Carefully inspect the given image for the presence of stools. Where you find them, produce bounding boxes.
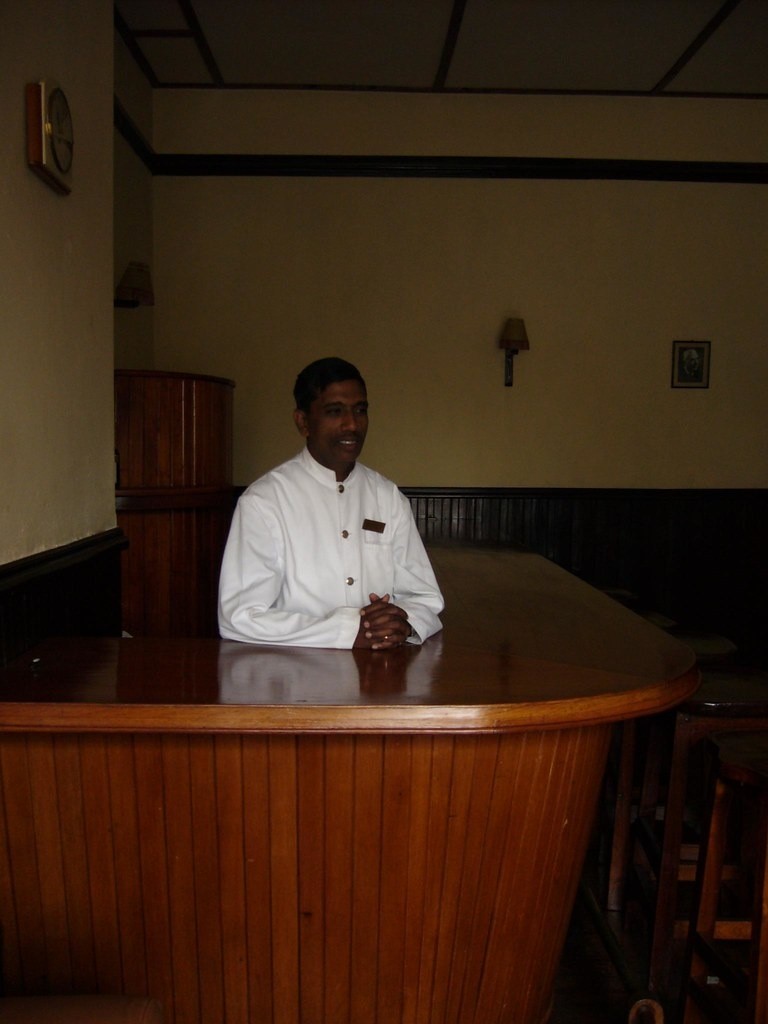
[600,587,768,1024]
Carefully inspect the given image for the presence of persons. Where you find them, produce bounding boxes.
[218,357,446,651]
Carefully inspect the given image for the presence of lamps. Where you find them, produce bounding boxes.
[114,261,155,310]
[499,317,529,386]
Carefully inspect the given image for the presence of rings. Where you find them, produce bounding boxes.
[384,636,388,639]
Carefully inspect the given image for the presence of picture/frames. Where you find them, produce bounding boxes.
[671,340,711,389]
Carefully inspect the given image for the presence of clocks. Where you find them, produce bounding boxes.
[24,77,75,197]
